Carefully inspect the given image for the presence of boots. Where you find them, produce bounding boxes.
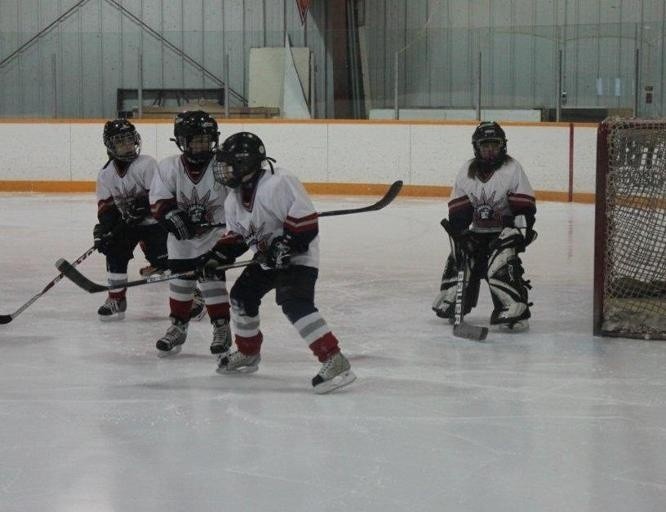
[156,314,188,351]
[211,320,231,354]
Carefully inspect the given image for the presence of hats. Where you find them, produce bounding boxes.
[471,120,507,164]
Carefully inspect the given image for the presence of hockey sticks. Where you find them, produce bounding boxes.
[55,258,258,293]
[200,180,402,229]
[453,255,488,341]
[0,248,95,323]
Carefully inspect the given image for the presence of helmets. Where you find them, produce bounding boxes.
[173,110,217,164]
[214,131,267,187]
[102,119,142,161]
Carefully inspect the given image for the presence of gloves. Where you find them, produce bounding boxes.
[157,205,196,242]
[266,231,297,267]
[194,247,229,284]
[92,224,116,251]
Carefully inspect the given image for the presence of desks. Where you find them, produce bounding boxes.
[132,105,280,119]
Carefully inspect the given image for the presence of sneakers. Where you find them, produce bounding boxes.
[311,346,351,387]
[97,295,126,317]
[217,351,263,370]
[187,288,204,317]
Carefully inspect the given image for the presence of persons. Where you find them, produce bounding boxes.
[430,120,538,330]
[194,129,351,387]
[147,108,237,354]
[92,118,204,318]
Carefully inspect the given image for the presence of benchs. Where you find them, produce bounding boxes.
[116,87,224,118]
